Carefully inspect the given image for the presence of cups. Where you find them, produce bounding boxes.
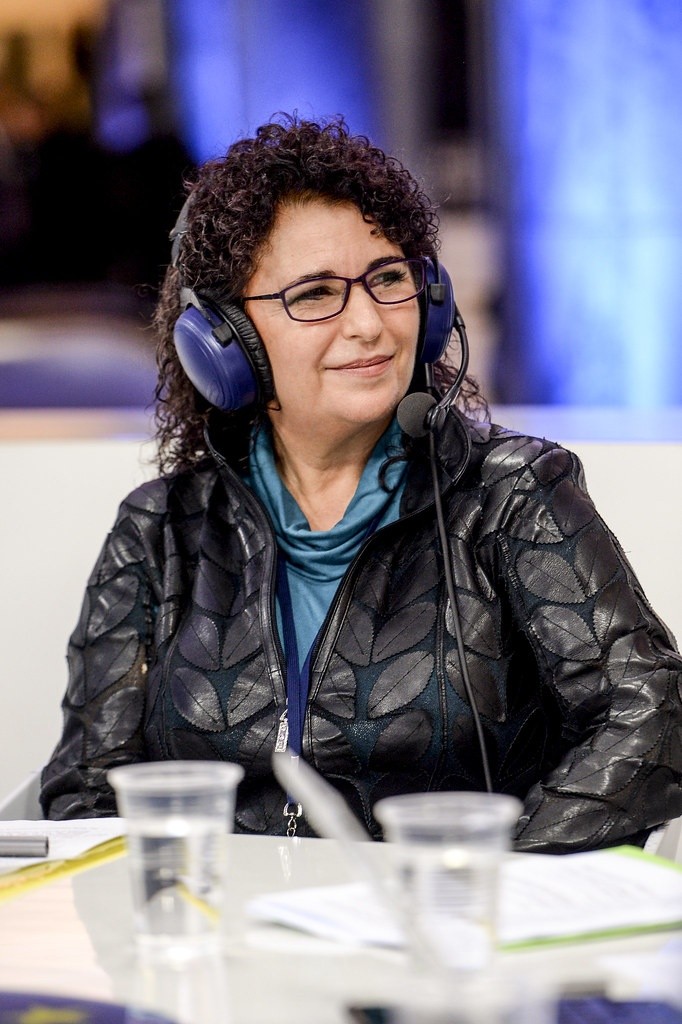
[375,792,524,989]
[106,763,243,948]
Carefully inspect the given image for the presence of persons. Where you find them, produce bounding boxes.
[37,103,682,855]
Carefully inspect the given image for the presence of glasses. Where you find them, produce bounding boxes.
[241,258,427,322]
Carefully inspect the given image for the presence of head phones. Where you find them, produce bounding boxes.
[171,171,466,421]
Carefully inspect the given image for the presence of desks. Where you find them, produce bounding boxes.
[0,834,682,1024]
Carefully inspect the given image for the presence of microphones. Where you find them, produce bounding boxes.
[396,327,468,436]
